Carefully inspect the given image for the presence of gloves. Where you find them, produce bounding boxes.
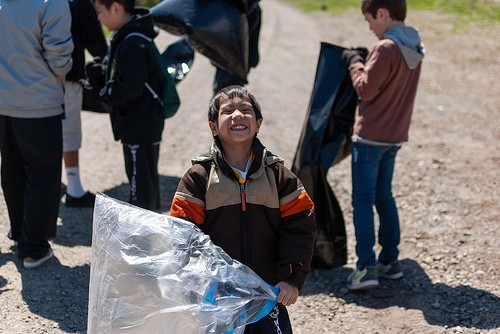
[342,46,369,66]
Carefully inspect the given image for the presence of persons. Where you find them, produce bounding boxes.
[1,0,180,269]
[170,85,316,334]
[342,0,425,291]
[213,0,262,95]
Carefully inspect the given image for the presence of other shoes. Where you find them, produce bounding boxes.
[23,247,54,268]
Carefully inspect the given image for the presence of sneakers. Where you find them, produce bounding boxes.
[347,266,379,290]
[376,260,404,280]
[65,191,97,207]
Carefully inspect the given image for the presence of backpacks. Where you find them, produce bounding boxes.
[124,32,181,119]
